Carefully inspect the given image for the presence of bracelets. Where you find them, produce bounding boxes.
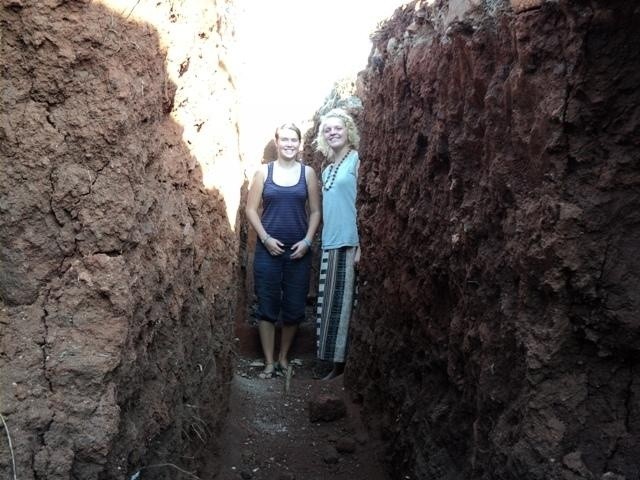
[303,236,313,247]
[258,234,271,244]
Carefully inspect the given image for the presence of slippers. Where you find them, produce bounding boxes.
[278,359,295,376]
[259,363,275,379]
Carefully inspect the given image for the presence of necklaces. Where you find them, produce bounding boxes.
[322,150,354,191]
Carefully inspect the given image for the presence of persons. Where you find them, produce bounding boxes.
[244,121,322,382]
[313,108,363,381]
